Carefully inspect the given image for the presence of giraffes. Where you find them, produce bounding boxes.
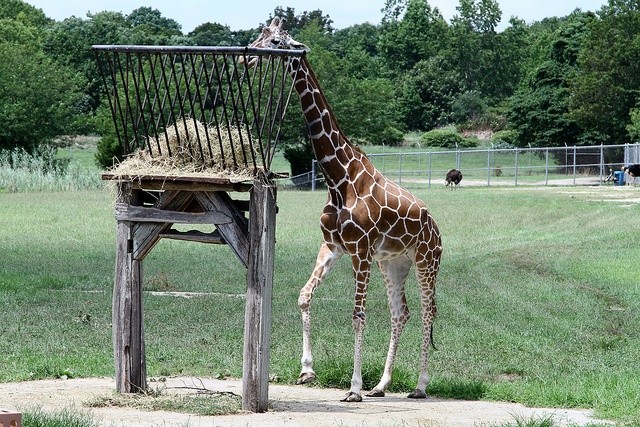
[233,16,443,402]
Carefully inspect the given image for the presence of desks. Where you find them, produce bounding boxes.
[101,174,279,413]
